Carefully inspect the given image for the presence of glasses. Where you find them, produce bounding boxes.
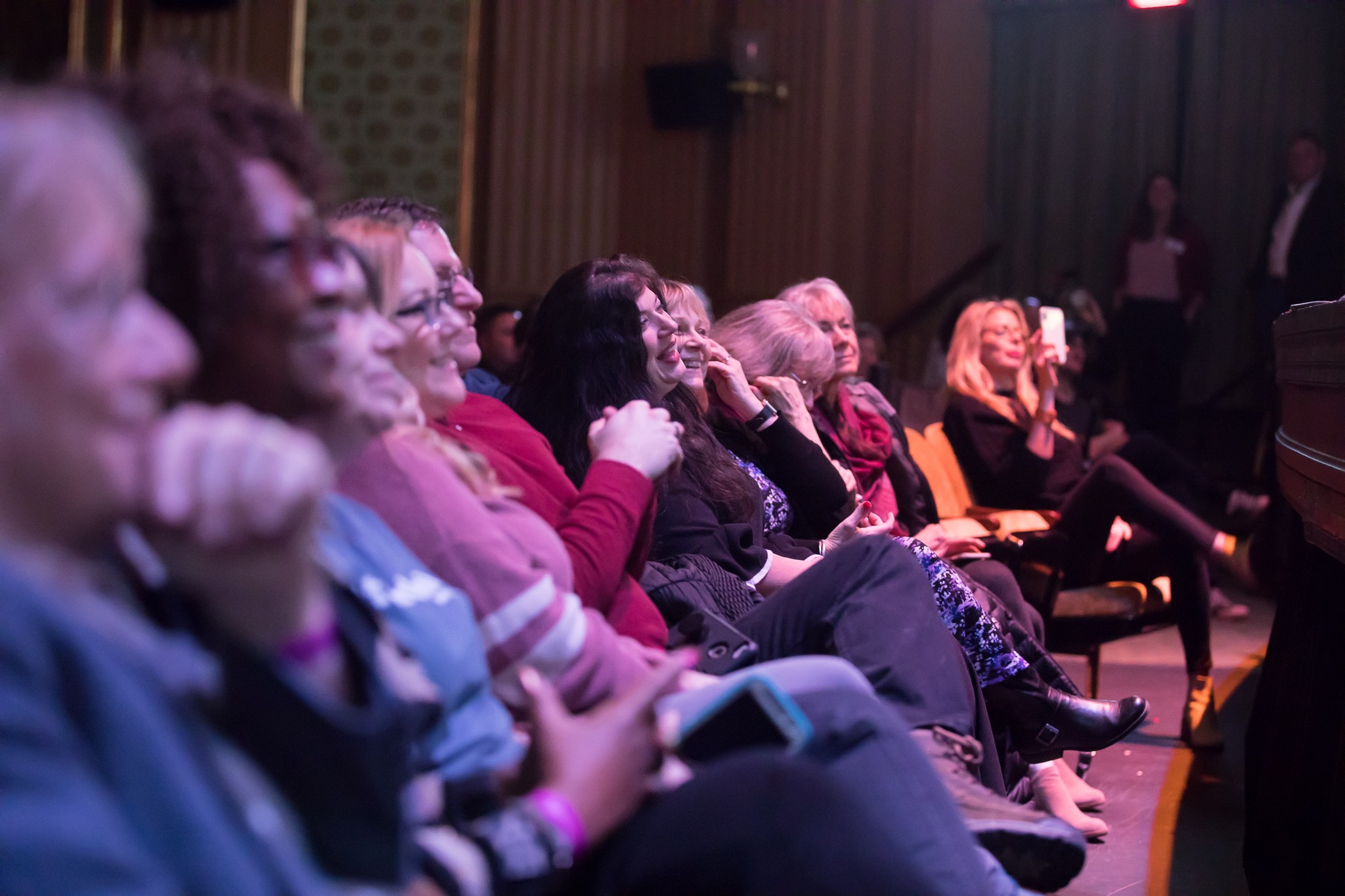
[790,371,822,401]
[394,285,455,326]
[256,202,339,271]
[438,263,474,289]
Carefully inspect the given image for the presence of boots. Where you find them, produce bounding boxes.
[996,669,1152,765]
[1028,758,1108,837]
[1213,533,1265,595]
[1181,673,1225,752]
[909,725,1087,895]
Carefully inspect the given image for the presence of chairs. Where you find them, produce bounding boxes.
[906,423,1178,746]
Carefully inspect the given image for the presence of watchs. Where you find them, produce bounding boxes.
[742,397,779,430]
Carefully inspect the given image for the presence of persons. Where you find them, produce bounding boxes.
[1,70,1345,895]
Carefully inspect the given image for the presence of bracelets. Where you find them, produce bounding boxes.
[819,539,827,557]
[530,786,596,863]
[1036,406,1057,424]
[245,603,344,669]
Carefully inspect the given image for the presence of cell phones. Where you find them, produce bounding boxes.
[669,674,814,767]
[1040,304,1067,366]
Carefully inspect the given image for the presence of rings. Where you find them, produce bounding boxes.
[670,421,683,437]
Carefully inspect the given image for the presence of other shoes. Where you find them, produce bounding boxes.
[1226,490,1271,516]
[1209,587,1249,620]
